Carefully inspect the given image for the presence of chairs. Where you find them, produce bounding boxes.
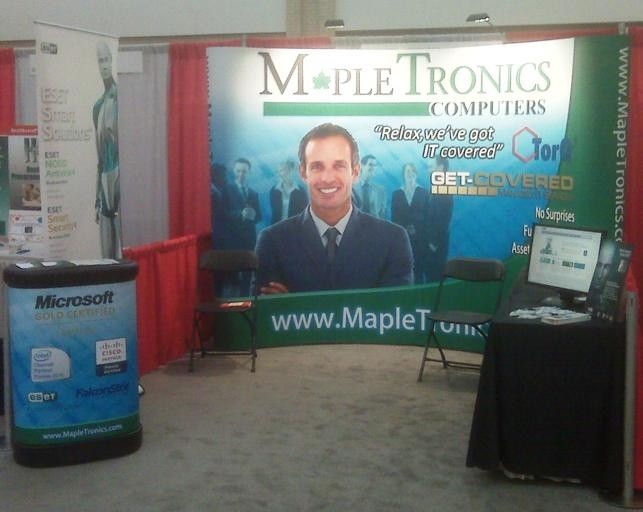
[187,250,259,371]
[417,257,506,381]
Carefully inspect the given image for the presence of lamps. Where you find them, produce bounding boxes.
[324,19,345,31]
[466,14,493,28]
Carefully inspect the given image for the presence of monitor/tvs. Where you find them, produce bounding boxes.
[524,222,607,313]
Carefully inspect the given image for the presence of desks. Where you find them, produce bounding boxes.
[464,264,626,485]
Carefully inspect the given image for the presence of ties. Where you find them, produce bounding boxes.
[363,183,370,213]
[241,187,248,199]
[324,228,340,264]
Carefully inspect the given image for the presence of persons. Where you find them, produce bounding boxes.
[212,162,230,191]
[584,239,619,319]
[248,122,415,297]
[421,154,453,284]
[92,41,124,262]
[268,157,308,225]
[224,158,262,298]
[210,151,239,298]
[352,154,387,219]
[21,137,41,201]
[391,162,428,283]
